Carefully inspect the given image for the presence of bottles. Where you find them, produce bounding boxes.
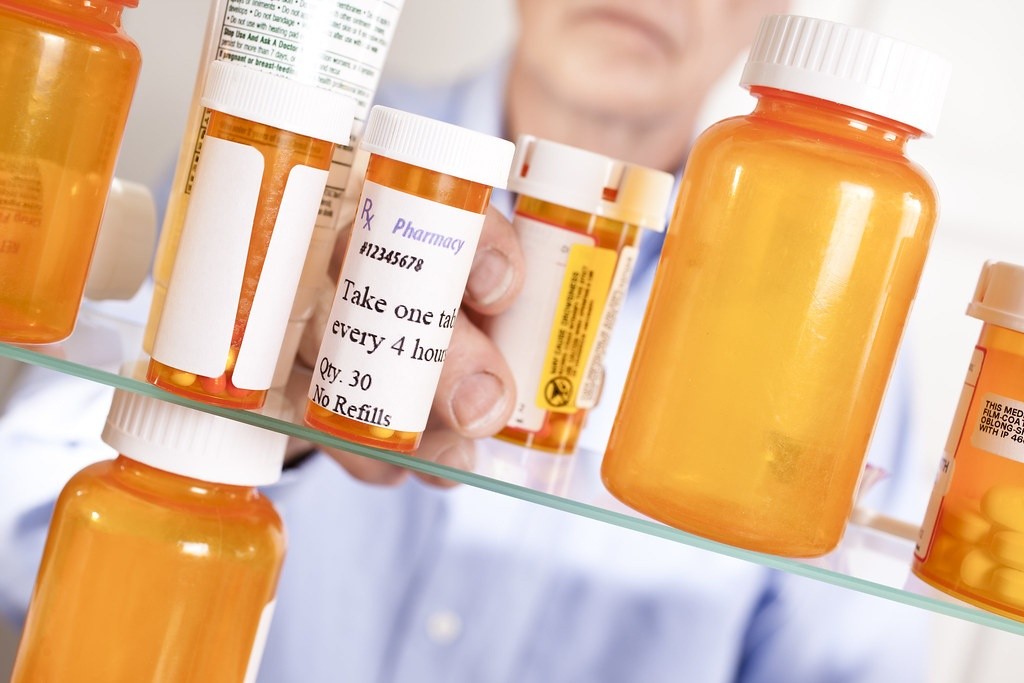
[912,257,1024,624]
[304,105,517,453]
[600,15,940,558]
[484,133,675,456]
[10,359,289,683]
[0,0,144,346]
[144,59,358,411]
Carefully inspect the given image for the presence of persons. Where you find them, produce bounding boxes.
[0,1,943,682]
